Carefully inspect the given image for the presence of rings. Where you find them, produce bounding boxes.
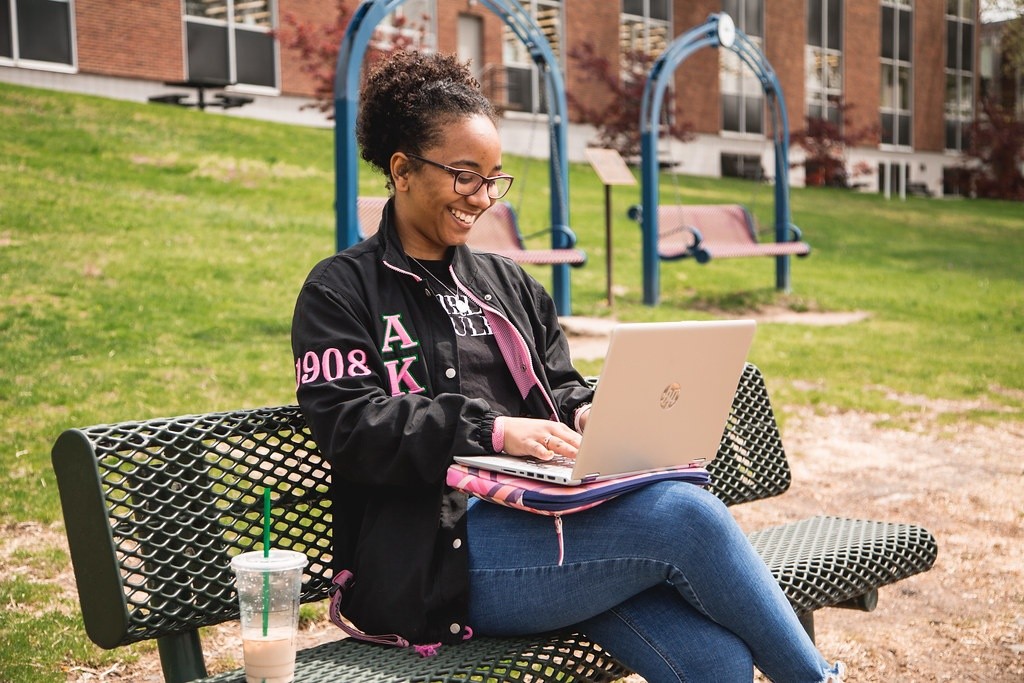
[544,435,553,448]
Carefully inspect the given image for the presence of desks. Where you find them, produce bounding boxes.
[165,77,229,112]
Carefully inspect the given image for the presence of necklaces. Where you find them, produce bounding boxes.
[405,250,469,313]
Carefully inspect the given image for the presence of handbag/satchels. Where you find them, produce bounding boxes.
[446,464,711,566]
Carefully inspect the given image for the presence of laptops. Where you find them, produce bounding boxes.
[453,319,756,485]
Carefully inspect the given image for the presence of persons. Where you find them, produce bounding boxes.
[292,49,845,683]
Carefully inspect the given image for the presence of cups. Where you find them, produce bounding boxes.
[231,549,309,683]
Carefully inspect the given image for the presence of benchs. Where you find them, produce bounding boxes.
[50,358,937,683]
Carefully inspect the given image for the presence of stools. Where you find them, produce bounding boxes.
[148,93,254,109]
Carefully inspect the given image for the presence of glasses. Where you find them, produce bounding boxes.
[403,152,515,200]
